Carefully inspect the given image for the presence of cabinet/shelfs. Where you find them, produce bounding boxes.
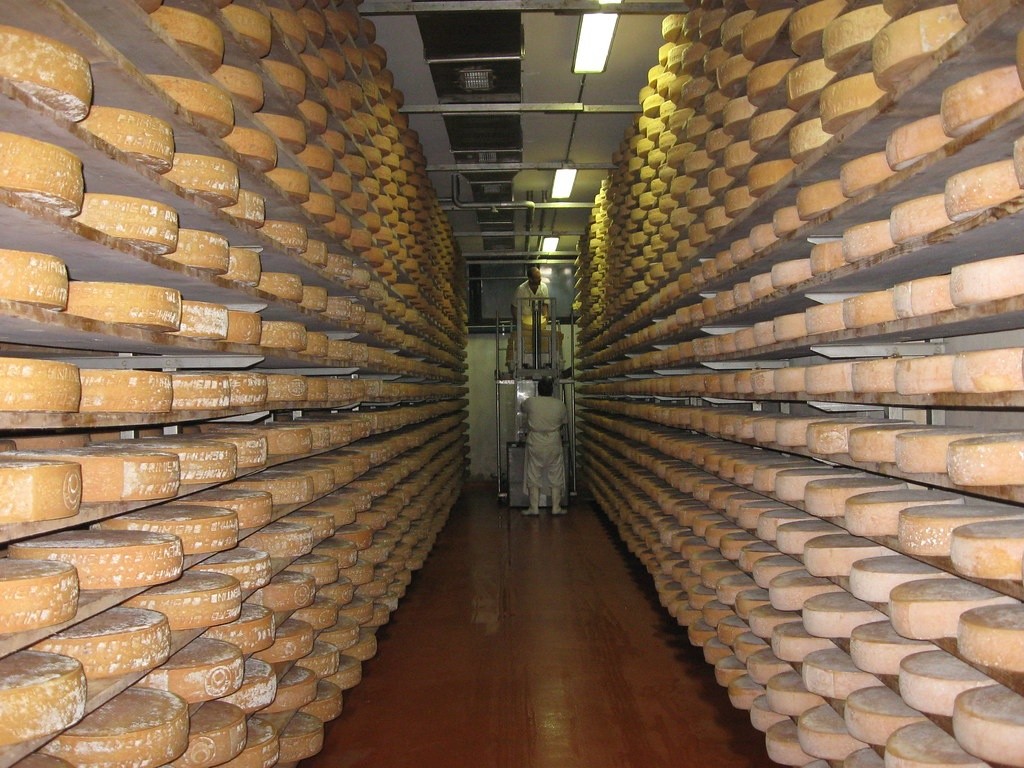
[572,0,1024,768]
[0,0,471,768]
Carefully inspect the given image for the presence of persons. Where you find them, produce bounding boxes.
[521,378,569,515]
[512,266,550,322]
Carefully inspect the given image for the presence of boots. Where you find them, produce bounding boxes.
[521,487,539,515]
[552,487,568,515]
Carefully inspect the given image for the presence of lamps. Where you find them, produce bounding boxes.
[543,236,560,253]
[550,168,577,200]
[571,12,620,74]
